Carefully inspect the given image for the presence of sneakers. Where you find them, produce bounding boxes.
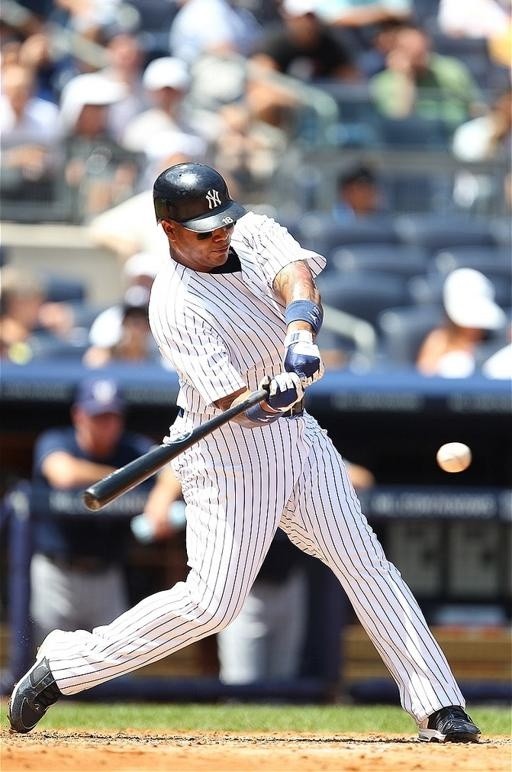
[418,706,482,743]
[9,629,65,734]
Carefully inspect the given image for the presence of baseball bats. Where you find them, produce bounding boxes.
[82,391,267,511]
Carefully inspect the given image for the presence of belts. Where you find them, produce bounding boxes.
[282,396,305,417]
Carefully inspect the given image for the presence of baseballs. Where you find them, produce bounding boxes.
[437,442,471,473]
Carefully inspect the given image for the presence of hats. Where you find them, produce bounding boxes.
[73,374,129,417]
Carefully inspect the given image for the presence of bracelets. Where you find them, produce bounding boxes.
[243,402,281,424]
[282,301,324,333]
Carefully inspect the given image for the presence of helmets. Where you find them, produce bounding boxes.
[152,161,248,233]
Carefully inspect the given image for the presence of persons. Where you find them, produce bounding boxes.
[1,3,510,379]
[217,455,374,687]
[7,163,482,740]
[26,376,179,655]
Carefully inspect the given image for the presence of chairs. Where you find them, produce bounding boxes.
[0,1,512,377]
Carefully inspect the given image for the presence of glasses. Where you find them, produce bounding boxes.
[197,219,236,240]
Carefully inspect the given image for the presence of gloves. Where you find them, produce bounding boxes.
[280,331,324,389]
[259,372,305,414]
